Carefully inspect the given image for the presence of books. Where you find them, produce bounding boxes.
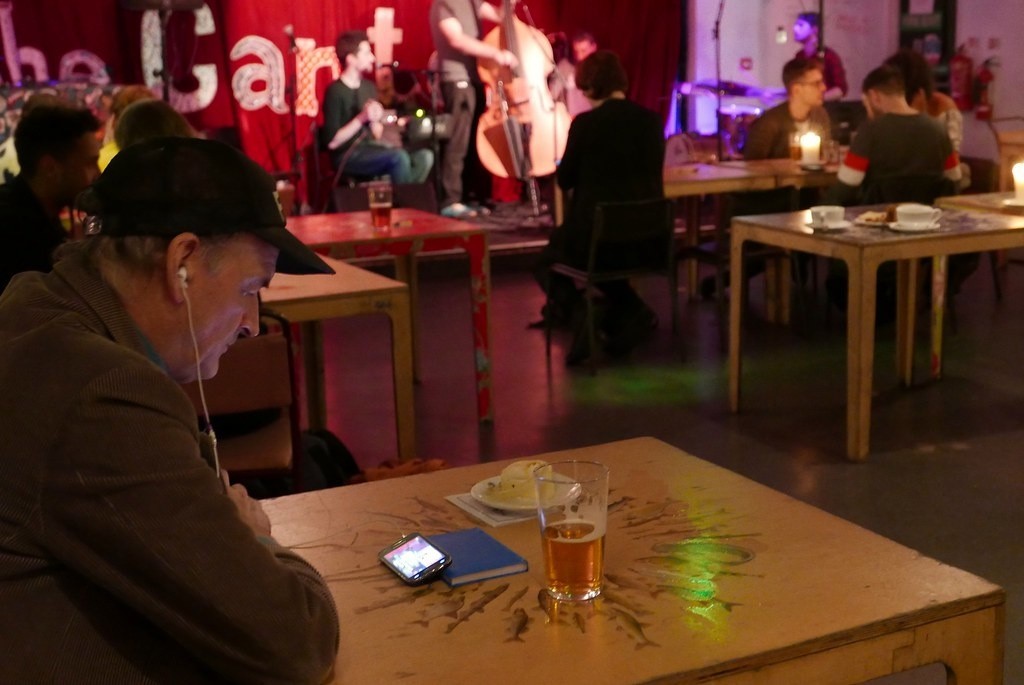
[427,528,529,588]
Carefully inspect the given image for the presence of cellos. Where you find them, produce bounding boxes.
[475,0,574,219]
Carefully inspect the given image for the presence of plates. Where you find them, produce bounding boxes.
[888,221,941,232]
[805,220,852,231]
[470,472,582,511]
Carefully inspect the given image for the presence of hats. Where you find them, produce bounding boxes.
[86,139,334,275]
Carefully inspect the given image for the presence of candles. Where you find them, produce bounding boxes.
[801,132,820,165]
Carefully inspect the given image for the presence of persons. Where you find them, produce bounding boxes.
[703,10,961,311]
[538,51,673,334]
[0,137,340,685]
[324,1,601,217]
[0,86,205,298]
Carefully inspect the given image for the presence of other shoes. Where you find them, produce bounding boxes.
[600,314,663,358]
[701,271,729,298]
[441,201,479,220]
[470,200,491,219]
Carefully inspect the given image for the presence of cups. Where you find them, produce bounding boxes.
[368,187,391,234]
[532,458,611,601]
[896,203,941,229]
[811,206,845,226]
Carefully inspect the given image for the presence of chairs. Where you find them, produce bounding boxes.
[547,197,683,372]
[702,156,1005,336]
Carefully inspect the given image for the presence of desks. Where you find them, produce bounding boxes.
[715,159,864,323]
[283,208,494,428]
[254,438,1006,685]
[259,253,416,460]
[554,162,779,333]
[930,194,1024,380]
[730,201,1024,462]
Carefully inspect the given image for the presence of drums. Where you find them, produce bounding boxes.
[716,105,762,158]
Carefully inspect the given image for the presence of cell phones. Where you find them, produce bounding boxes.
[377,531,453,586]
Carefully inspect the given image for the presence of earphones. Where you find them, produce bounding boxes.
[177,265,188,288]
